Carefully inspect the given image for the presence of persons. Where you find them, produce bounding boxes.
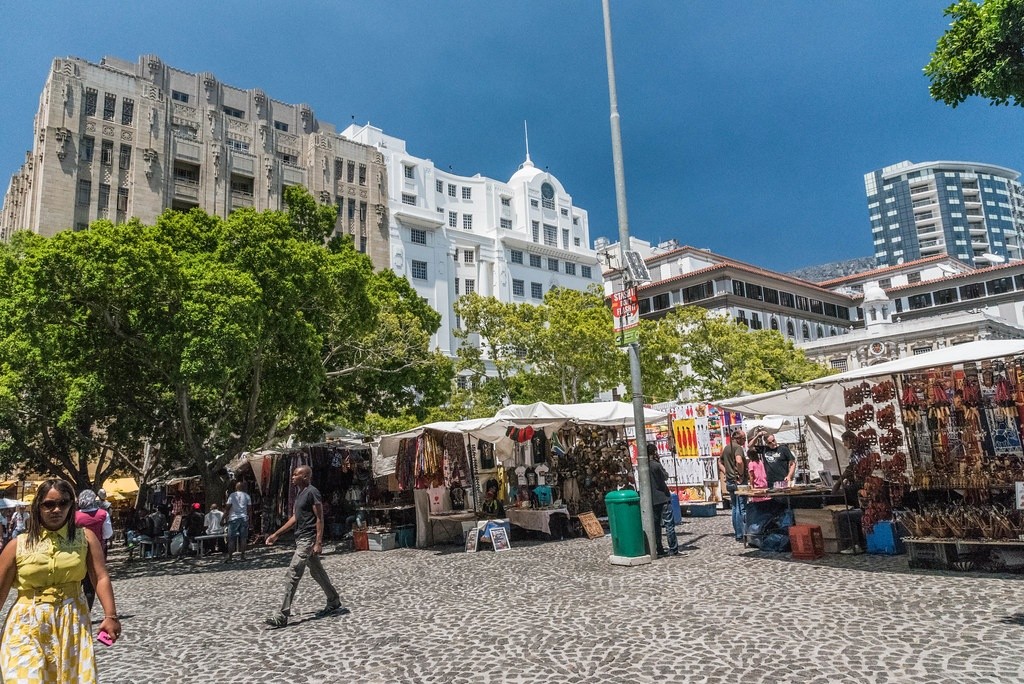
[832,430,867,503]
[265,466,342,624]
[149,503,205,559]
[476,489,505,519]
[646,444,688,556]
[75,489,114,611]
[748,450,771,504]
[0,480,122,684]
[0,509,11,548]
[719,431,748,541]
[96,489,112,517]
[9,505,30,539]
[204,503,227,552]
[747,431,796,486]
[220,482,253,562]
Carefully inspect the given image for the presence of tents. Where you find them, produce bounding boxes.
[711,339,1024,552]
[241,400,667,548]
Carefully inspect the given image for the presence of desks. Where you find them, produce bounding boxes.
[733,489,831,548]
[506,508,570,540]
[428,512,475,546]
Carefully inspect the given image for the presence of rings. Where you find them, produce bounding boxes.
[117,633,119,635]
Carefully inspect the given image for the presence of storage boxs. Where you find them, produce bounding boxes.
[865,521,907,555]
[352,526,415,551]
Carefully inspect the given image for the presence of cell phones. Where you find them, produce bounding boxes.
[97,631,113,646]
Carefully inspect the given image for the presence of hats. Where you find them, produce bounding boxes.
[192,503,200,509]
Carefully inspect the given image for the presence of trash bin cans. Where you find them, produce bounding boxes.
[605,490,646,558]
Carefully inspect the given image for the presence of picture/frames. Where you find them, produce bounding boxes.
[489,527,511,552]
[465,527,478,553]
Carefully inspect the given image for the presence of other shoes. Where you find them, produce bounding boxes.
[657,552,670,557]
[840,546,863,554]
[264,615,288,626]
[737,536,747,541]
[670,552,689,558]
[315,599,342,618]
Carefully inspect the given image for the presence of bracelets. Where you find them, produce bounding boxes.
[105,615,118,618]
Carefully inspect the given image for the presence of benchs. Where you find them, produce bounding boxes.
[194,533,239,559]
[139,536,169,561]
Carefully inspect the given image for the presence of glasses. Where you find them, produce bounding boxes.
[769,439,775,444]
[40,498,72,510]
